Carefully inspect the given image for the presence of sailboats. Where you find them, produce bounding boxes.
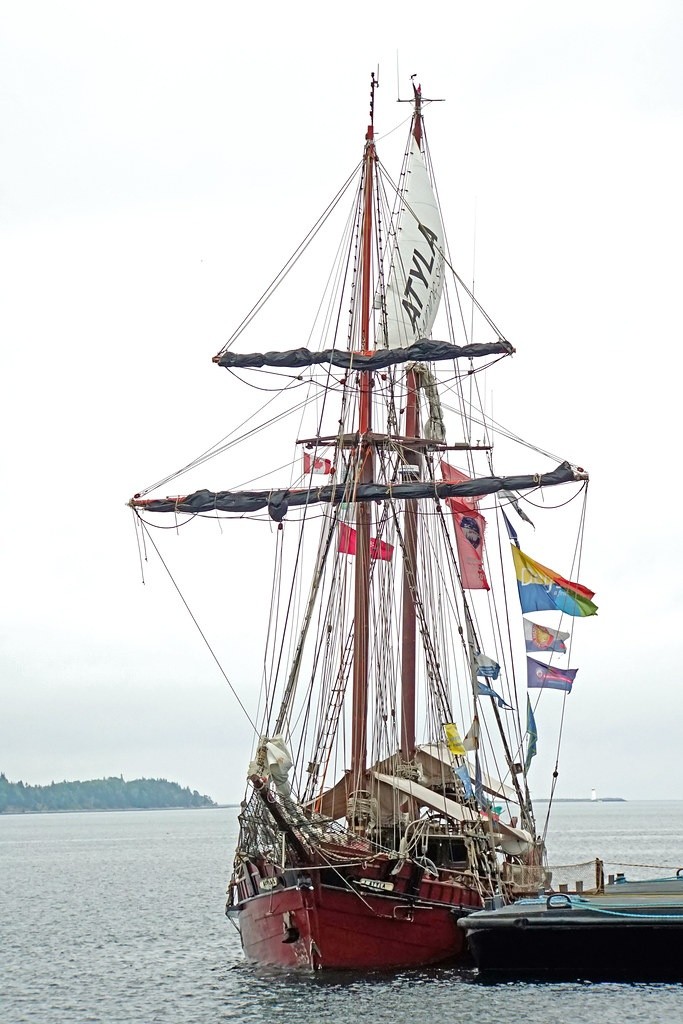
[125,50,598,975]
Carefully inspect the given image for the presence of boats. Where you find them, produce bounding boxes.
[458,861,683,982]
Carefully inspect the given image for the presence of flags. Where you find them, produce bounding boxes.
[497,487,535,528]
[473,649,500,680]
[527,656,579,695]
[370,538,394,562]
[441,460,490,591]
[524,692,538,778]
[510,543,599,617]
[304,452,332,475]
[337,522,357,555]
[463,712,480,751]
[499,501,520,550]
[445,723,465,754]
[475,750,491,809]
[477,681,514,711]
[523,617,570,653]
[453,764,472,799]
